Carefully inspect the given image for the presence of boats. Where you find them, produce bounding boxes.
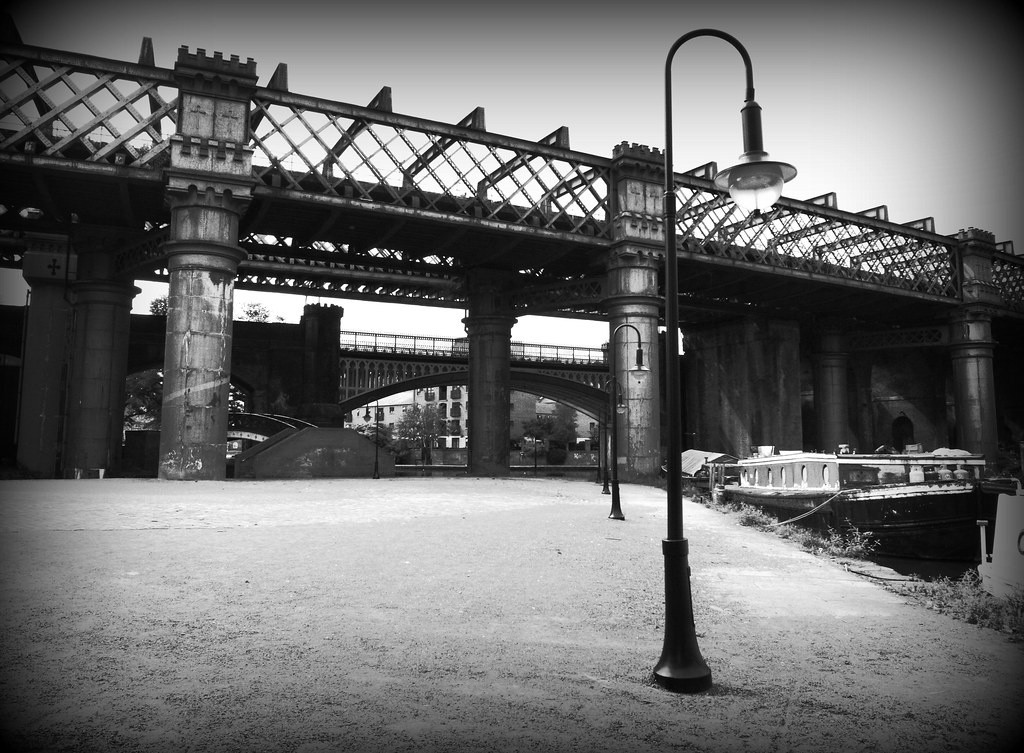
[703,442,992,561]
[659,448,743,492]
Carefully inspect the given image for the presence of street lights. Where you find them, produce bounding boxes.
[650,24,799,695]
[601,379,629,496]
[595,403,611,484]
[610,323,651,524]
[363,397,380,479]
